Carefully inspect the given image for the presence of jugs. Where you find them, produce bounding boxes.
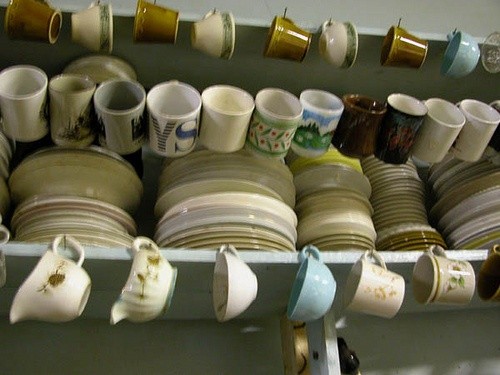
[10,235,93,324]
[109,236,179,325]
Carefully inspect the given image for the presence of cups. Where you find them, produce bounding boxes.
[144,79,202,159]
[332,93,387,159]
[47,72,97,150]
[440,30,481,79]
[200,83,255,154]
[411,97,467,164]
[318,20,359,72]
[4,0,63,44]
[244,86,304,161]
[214,245,500,322]
[132,0,180,47]
[191,9,236,63]
[375,93,429,165]
[262,15,313,64]
[454,98,500,163]
[93,77,146,156]
[291,88,345,161]
[0,64,48,143]
[71,1,113,56]
[481,32,500,73]
[380,25,429,71]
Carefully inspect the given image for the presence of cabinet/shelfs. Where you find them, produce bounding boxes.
[0,0,500,375]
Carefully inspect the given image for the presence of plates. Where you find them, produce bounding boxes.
[63,56,137,87]
[152,147,500,252]
[0,119,143,250]
